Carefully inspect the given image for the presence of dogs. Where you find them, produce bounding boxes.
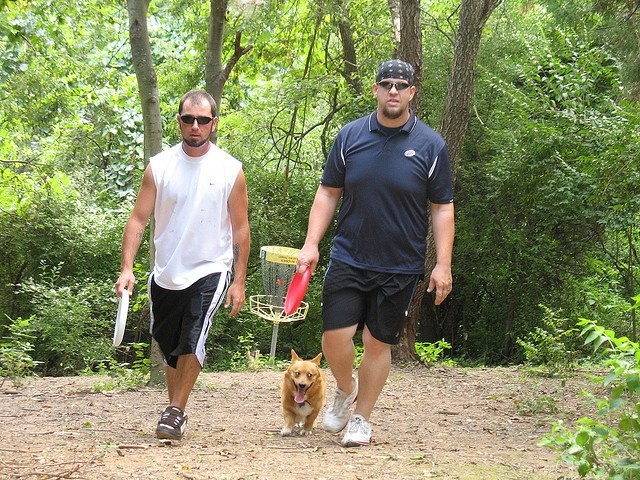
[280,348,325,437]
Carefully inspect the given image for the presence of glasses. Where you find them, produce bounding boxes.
[376,81,411,90]
[180,115,214,125]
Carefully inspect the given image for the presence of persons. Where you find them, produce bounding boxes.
[296,59,454,446]
[116,89,250,438]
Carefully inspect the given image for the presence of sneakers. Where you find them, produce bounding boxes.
[341,414,373,447]
[156,405,188,440]
[323,374,359,434]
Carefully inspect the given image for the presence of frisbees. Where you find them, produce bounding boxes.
[284,267,310,315]
[113,290,129,346]
